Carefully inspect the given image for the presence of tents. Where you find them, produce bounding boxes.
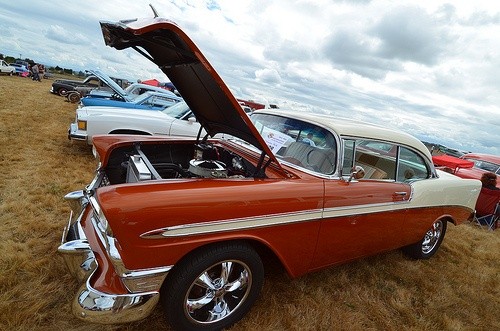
[142,79,174,89]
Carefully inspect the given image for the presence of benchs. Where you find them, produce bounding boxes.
[283,142,388,179]
[345,149,413,182]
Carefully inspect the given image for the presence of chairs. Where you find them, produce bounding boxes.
[472,187,500,231]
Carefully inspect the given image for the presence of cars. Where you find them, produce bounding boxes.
[50,68,252,152]
[0,59,16,77]
[433,152,500,228]
[56,2,483,331]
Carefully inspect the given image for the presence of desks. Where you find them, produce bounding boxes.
[431,156,474,177]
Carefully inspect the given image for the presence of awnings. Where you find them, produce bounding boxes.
[432,155,474,174]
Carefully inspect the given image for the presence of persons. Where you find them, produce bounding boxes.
[32,63,42,82]
